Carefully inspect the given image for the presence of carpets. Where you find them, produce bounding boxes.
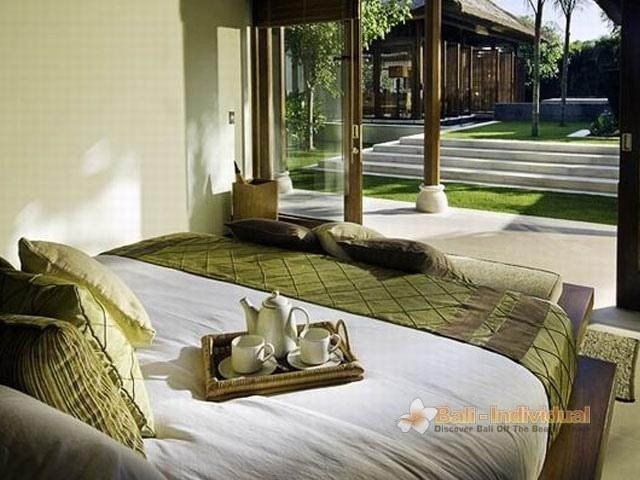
[579,328,640,403]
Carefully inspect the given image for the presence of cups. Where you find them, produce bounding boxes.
[231,334,276,374]
[299,326,342,365]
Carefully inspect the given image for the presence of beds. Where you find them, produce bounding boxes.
[0,231,578,480]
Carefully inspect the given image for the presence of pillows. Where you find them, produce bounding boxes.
[17,237,155,350]
[0,257,156,438]
[1,313,146,459]
[0,385,167,480]
[223,219,473,283]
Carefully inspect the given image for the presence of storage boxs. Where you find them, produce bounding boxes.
[232,178,279,222]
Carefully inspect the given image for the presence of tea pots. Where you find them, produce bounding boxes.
[239,288,311,360]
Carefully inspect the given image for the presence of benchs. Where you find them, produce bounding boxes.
[448,253,594,344]
[537,353,617,479]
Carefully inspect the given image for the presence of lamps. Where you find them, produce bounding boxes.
[388,64,409,120]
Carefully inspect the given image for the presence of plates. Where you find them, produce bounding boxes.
[286,345,343,370]
[218,355,278,379]
